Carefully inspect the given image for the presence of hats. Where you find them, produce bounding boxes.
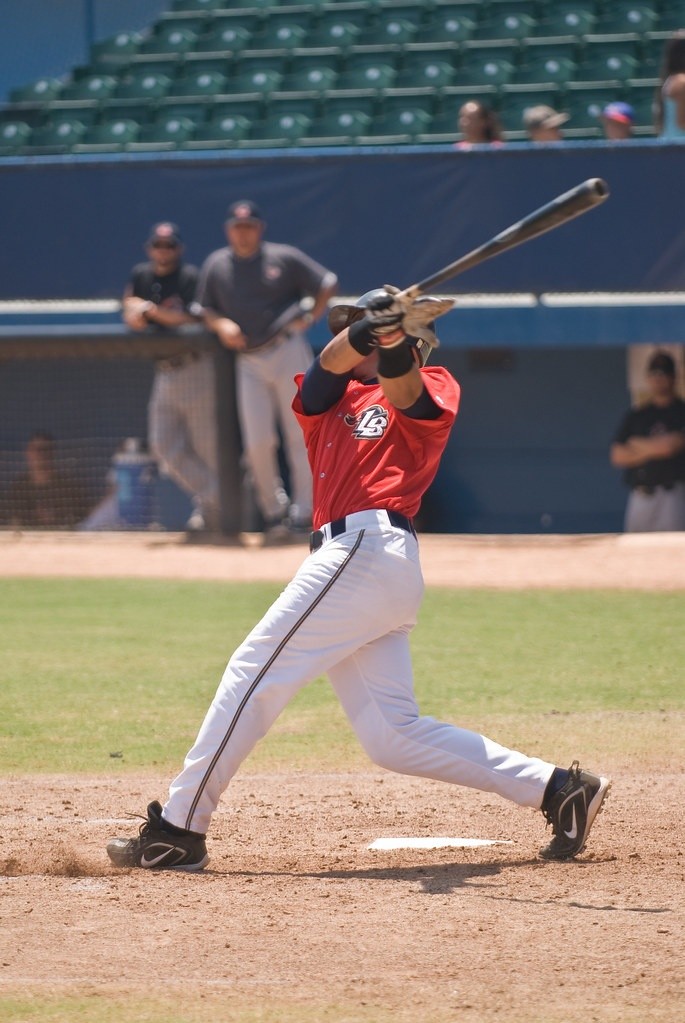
[151,225,179,245]
[599,103,632,125]
[525,106,563,129]
[226,201,261,226]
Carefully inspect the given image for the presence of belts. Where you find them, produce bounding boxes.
[309,510,417,551]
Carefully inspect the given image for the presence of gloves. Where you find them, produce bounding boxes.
[357,283,455,348]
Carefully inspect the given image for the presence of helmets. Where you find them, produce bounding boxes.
[326,288,436,368]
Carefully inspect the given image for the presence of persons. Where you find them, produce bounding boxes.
[524,104,569,139]
[121,221,219,532]
[454,99,502,144]
[656,32,685,138]
[596,102,635,139]
[191,201,338,535]
[106,285,610,870]
[610,352,685,533]
[0,431,112,529]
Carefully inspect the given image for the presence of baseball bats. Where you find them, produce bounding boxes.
[394,177,611,299]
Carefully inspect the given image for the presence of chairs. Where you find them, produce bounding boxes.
[0,0,685,157]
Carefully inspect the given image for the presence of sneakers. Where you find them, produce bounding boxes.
[106,799,211,871]
[537,760,613,859]
